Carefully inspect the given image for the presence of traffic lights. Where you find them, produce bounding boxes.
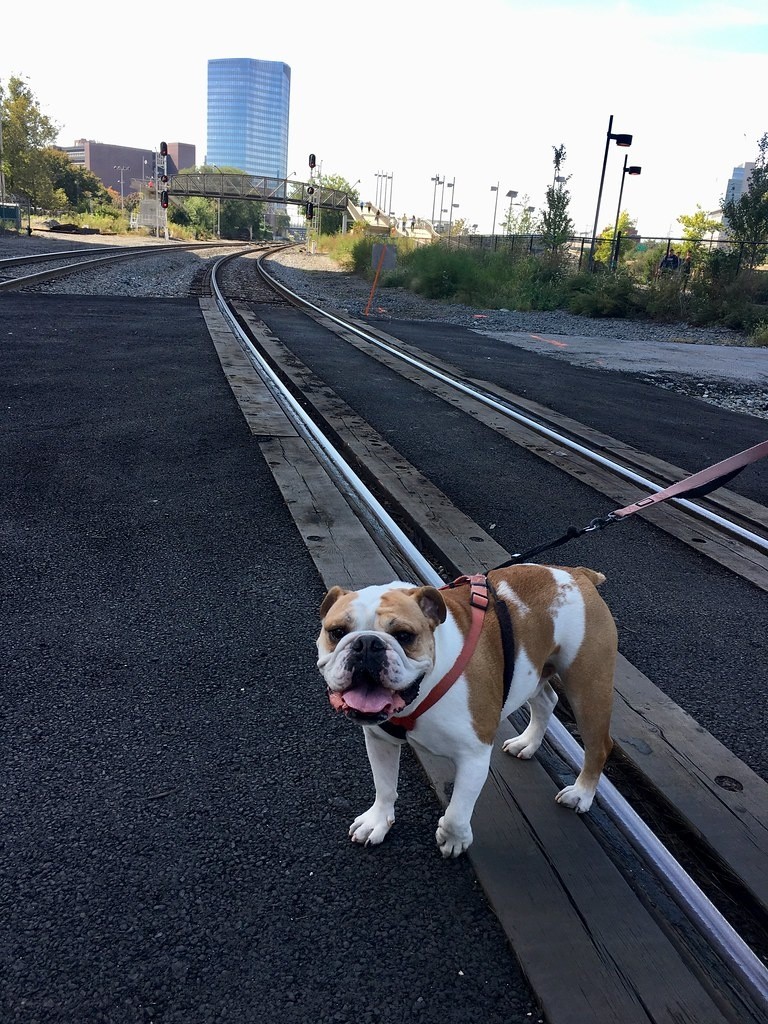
[160,141,167,156]
[305,202,313,219]
[161,190,168,208]
[309,154,316,168]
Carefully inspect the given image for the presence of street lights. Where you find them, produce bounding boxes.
[588,112,634,272]
[609,154,642,272]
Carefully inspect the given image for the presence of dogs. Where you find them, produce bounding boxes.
[314,564,620,855]
